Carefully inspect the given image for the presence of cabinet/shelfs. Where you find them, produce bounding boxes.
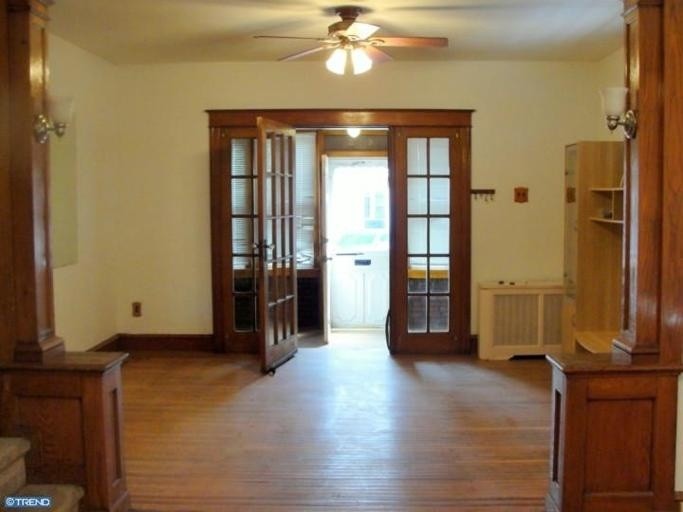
[477,281,562,361]
[561,141,622,355]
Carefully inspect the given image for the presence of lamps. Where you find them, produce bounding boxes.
[34,96,74,145]
[599,86,636,140]
[326,42,372,76]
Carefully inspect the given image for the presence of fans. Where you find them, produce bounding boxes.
[253,8,448,64]
